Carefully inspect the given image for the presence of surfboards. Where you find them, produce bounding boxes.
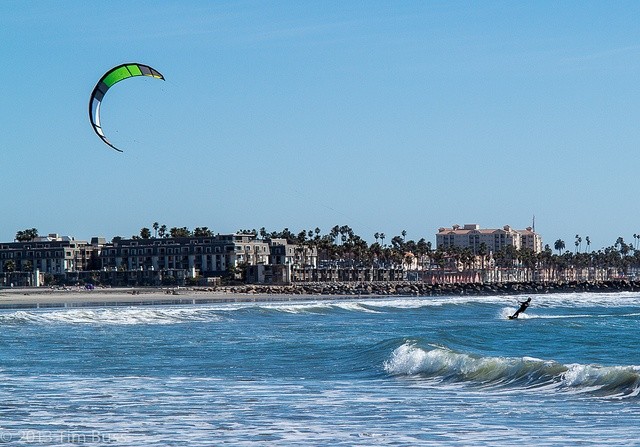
[508,316,516,319]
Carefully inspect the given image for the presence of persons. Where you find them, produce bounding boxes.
[508,297,532,319]
